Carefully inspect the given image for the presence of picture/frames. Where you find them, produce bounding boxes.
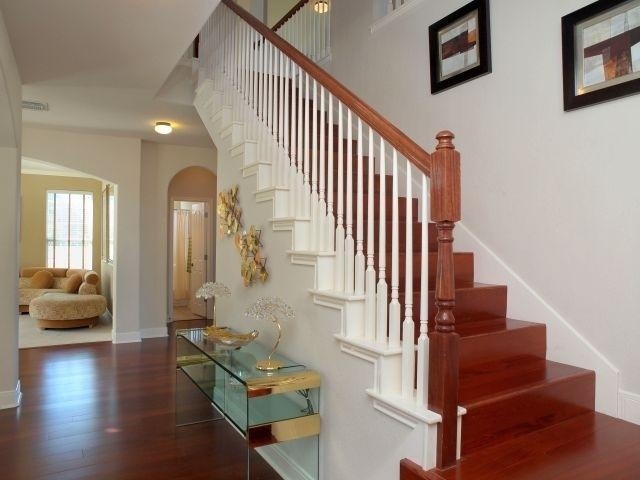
[561,0,640,112]
[428,0,492,96]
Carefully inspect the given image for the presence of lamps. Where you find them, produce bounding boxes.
[314,1,328,13]
[154,122,172,135]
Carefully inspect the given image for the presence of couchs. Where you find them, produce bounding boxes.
[19,267,106,330]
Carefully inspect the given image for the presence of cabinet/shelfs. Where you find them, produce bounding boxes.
[175,327,321,480]
